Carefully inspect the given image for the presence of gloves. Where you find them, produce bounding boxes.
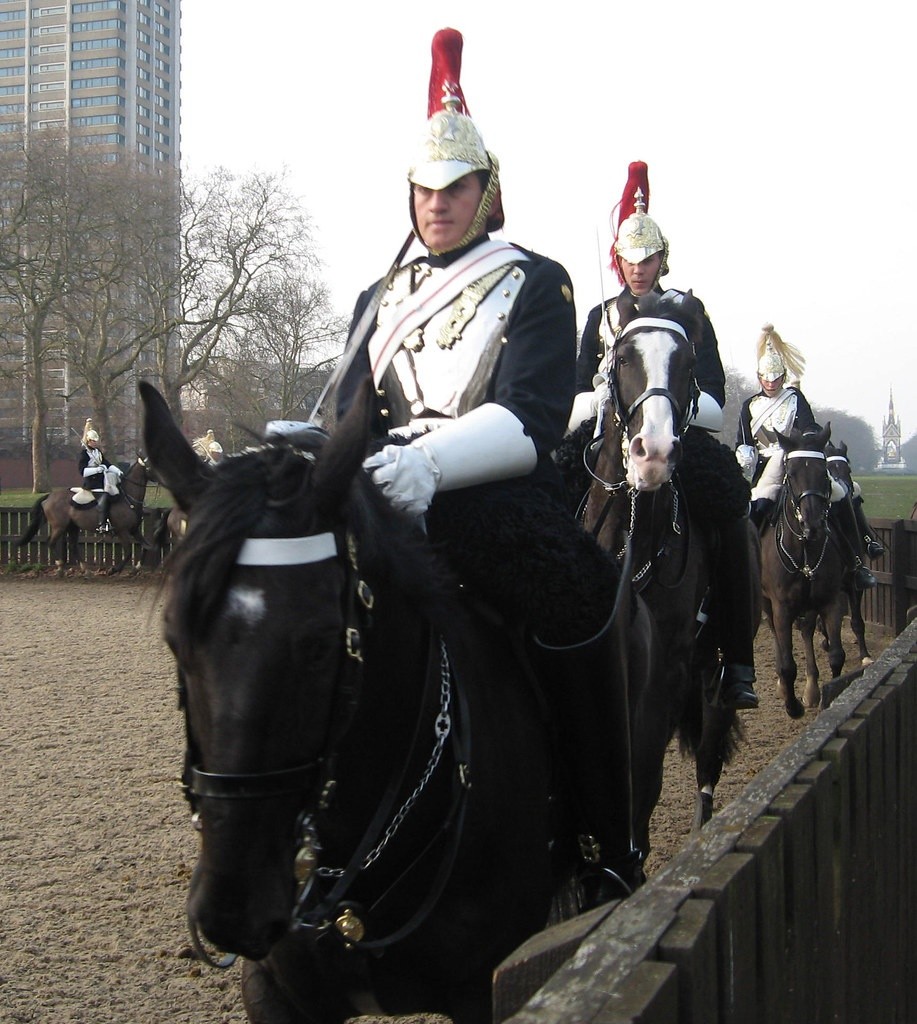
[736,444,754,467]
[83,467,104,478]
[362,403,538,517]
[108,465,123,476]
[569,379,608,431]
[683,388,723,433]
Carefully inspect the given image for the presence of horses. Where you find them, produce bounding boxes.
[17,377,873,1022]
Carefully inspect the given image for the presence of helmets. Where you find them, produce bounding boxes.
[81,418,100,446]
[193,428,224,457]
[406,28,505,255]
[757,327,804,397]
[604,162,669,298]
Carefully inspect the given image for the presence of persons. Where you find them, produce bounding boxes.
[203,428,223,467]
[551,188,761,710]
[309,107,593,887]
[733,323,886,590]
[78,416,124,535]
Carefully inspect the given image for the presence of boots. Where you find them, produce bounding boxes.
[703,503,758,711]
[535,611,647,912]
[852,495,885,558]
[93,492,114,533]
[831,495,876,590]
[751,497,774,523]
